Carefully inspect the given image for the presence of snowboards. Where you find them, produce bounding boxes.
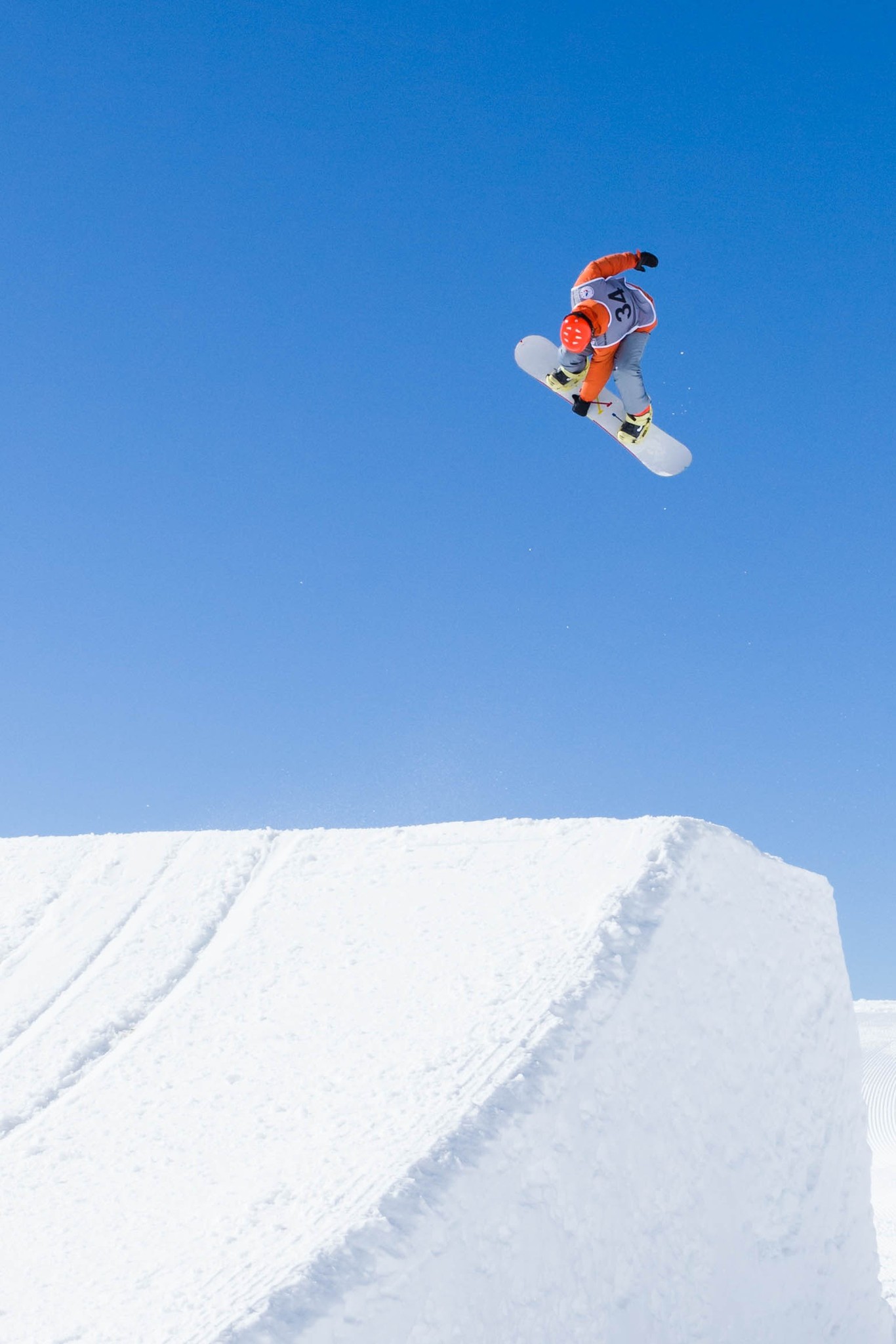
[515,335,692,477]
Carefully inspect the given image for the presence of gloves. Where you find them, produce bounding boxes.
[570,394,590,417]
[635,252,658,273]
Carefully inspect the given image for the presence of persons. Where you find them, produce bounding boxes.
[546,249,660,445]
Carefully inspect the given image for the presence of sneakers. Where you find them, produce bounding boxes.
[547,359,590,391]
[616,404,653,446]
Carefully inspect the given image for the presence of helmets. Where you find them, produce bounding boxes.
[560,313,592,354]
